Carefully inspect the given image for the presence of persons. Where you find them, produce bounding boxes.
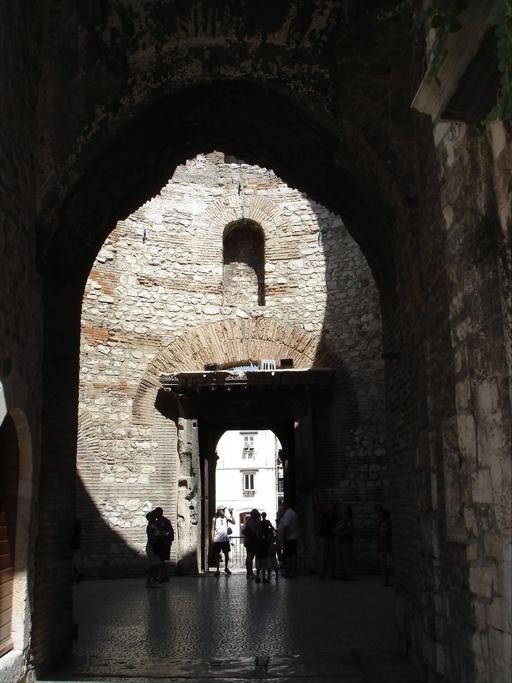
[146,507,174,588]
[242,500,300,584]
[211,505,235,576]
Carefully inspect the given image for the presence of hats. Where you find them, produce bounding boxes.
[215,505,225,510]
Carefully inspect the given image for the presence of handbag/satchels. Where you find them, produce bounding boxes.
[227,527,232,535]
